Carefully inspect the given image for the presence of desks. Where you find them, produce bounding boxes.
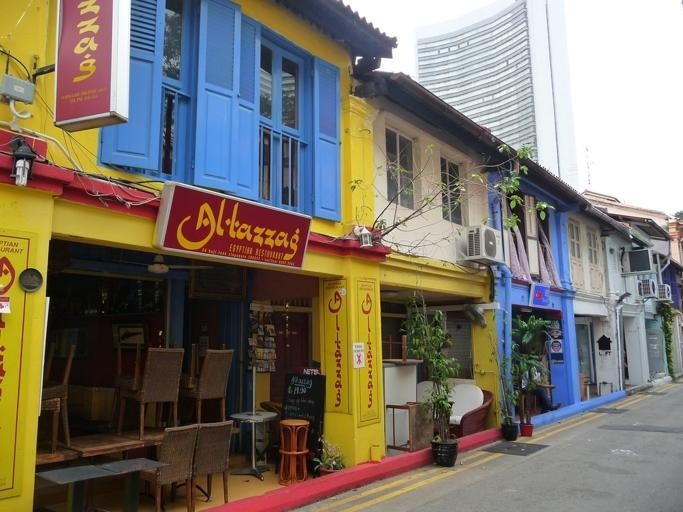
[386,401,434,453]
[382,335,424,366]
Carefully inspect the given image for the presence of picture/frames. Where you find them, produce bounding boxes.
[113,322,150,352]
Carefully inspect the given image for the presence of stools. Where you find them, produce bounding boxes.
[278,419,310,486]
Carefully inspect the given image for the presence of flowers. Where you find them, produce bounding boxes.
[313,435,343,470]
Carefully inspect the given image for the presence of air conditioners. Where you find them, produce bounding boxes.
[641,278,672,300]
[466,226,505,266]
[622,249,652,274]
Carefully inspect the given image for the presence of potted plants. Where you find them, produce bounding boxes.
[508,315,552,437]
[488,332,518,441]
[402,292,468,467]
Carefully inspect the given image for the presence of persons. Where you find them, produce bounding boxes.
[549,329,563,352]
[520,361,562,414]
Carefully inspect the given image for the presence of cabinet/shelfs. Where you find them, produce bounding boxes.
[383,363,417,447]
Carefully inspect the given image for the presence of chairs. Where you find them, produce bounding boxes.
[435,378,494,436]
[32,338,234,512]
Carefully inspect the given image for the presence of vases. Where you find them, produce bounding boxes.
[317,466,333,476]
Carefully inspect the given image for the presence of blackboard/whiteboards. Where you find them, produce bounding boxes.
[281,373,326,438]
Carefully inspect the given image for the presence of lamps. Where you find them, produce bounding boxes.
[9,134,37,189]
[354,225,374,248]
